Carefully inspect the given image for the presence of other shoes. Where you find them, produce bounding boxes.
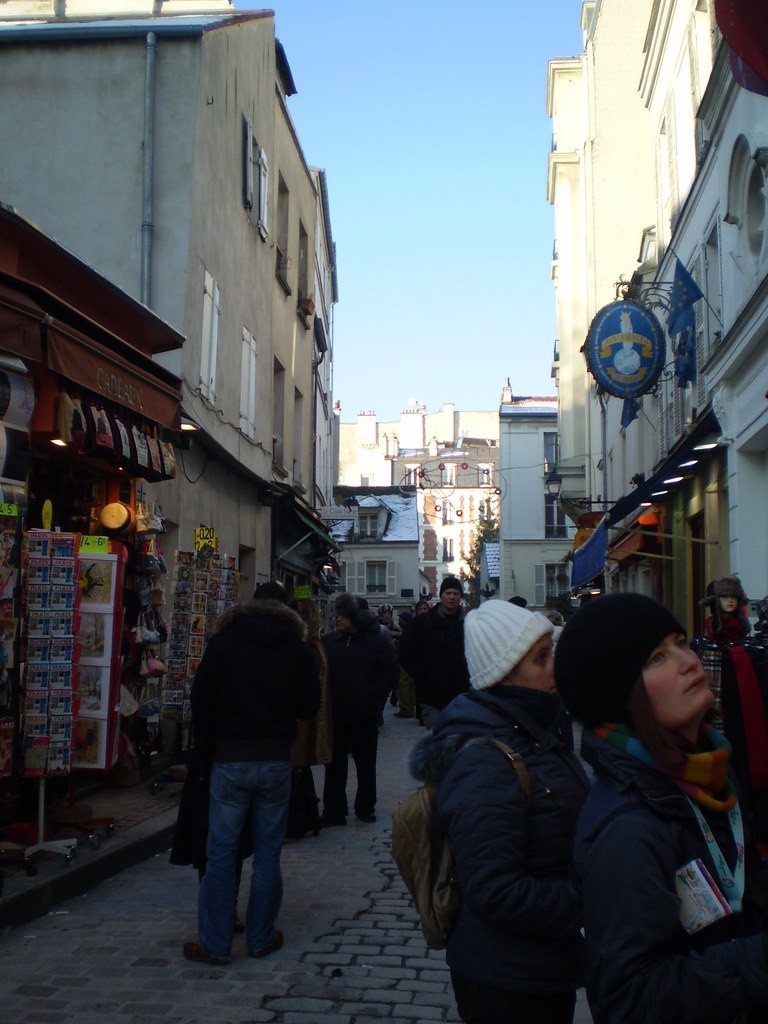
[182,942,231,965]
[248,929,284,958]
[355,812,377,824]
[317,815,348,827]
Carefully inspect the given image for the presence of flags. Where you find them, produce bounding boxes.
[665,261,706,337]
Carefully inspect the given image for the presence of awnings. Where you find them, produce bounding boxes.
[277,509,343,559]
[571,439,718,588]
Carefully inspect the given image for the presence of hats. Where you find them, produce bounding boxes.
[698,577,751,634]
[463,599,555,690]
[378,603,393,614]
[554,593,689,731]
[439,576,463,596]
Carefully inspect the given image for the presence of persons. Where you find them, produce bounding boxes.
[181,579,321,969]
[410,593,591,1024]
[170,597,320,932]
[554,592,768,1024]
[704,575,751,648]
[320,594,401,825]
[374,576,596,730]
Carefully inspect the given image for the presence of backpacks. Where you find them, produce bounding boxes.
[388,736,530,952]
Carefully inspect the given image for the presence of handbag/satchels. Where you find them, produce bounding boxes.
[114,499,168,762]
